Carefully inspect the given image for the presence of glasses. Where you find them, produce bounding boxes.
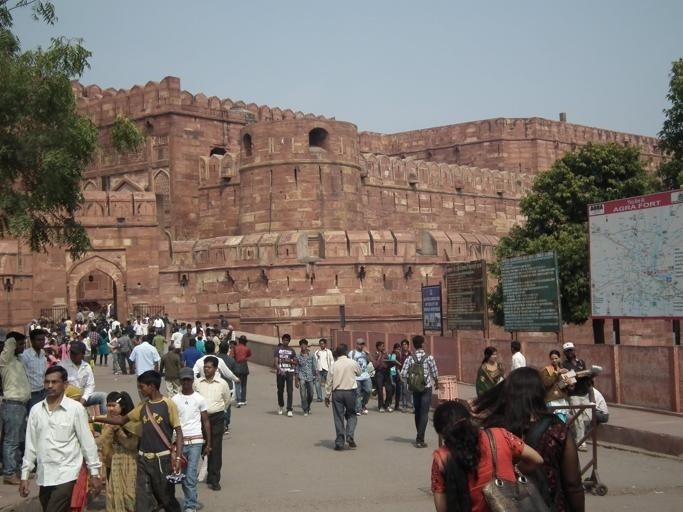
[202,365,216,371]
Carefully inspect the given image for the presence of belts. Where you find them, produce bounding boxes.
[30,388,46,397]
[1,398,28,408]
[183,438,207,449]
[137,449,171,461]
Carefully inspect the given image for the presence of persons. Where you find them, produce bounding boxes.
[399,336,439,447]
[431,341,609,512]
[273,333,416,417]
[324,344,362,450]
[0,303,253,512]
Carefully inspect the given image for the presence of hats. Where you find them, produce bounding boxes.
[560,340,575,350]
[355,338,365,346]
[67,340,86,354]
[178,366,195,381]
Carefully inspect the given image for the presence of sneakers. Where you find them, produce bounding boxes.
[277,406,283,415]
[334,438,347,450]
[303,410,311,418]
[362,407,369,415]
[344,436,357,448]
[287,410,293,417]
[3,471,36,487]
[209,481,222,491]
[234,400,248,409]
[356,412,361,416]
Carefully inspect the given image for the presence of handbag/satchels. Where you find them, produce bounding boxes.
[171,440,189,474]
[478,425,553,512]
